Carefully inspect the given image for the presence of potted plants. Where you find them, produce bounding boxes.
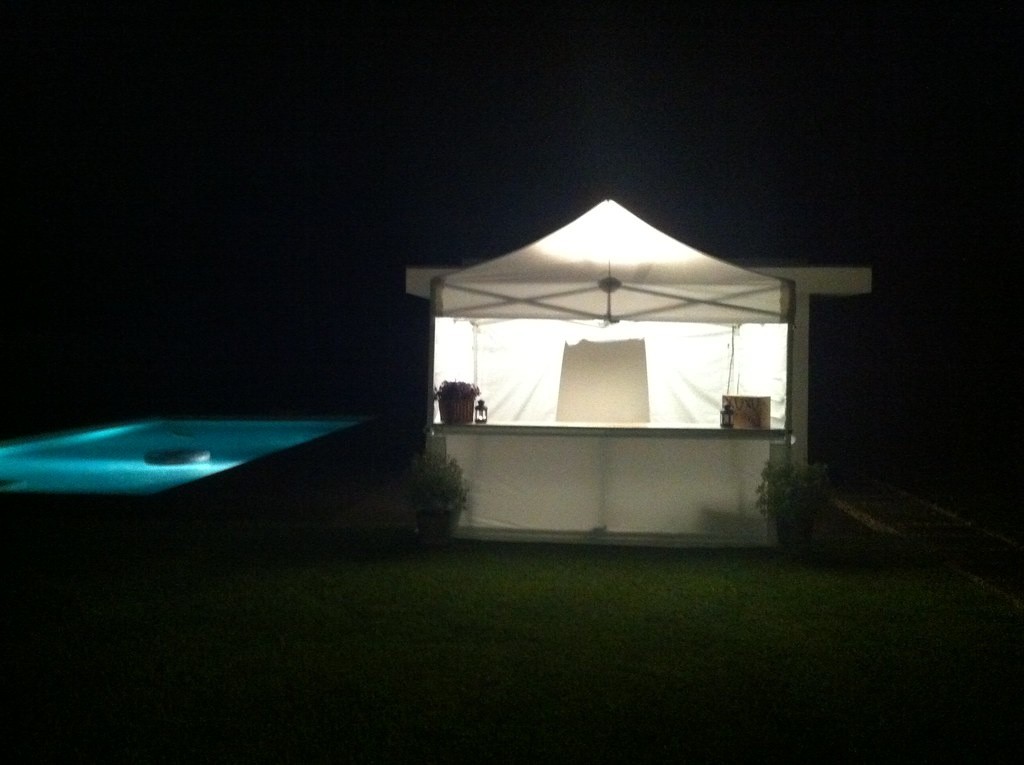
[433,381,480,424]
[407,452,470,550]
[756,461,828,551]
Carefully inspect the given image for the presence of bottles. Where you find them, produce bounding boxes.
[474,400,488,424]
[720,403,734,428]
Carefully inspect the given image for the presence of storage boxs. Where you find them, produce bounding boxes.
[721,395,772,430]
